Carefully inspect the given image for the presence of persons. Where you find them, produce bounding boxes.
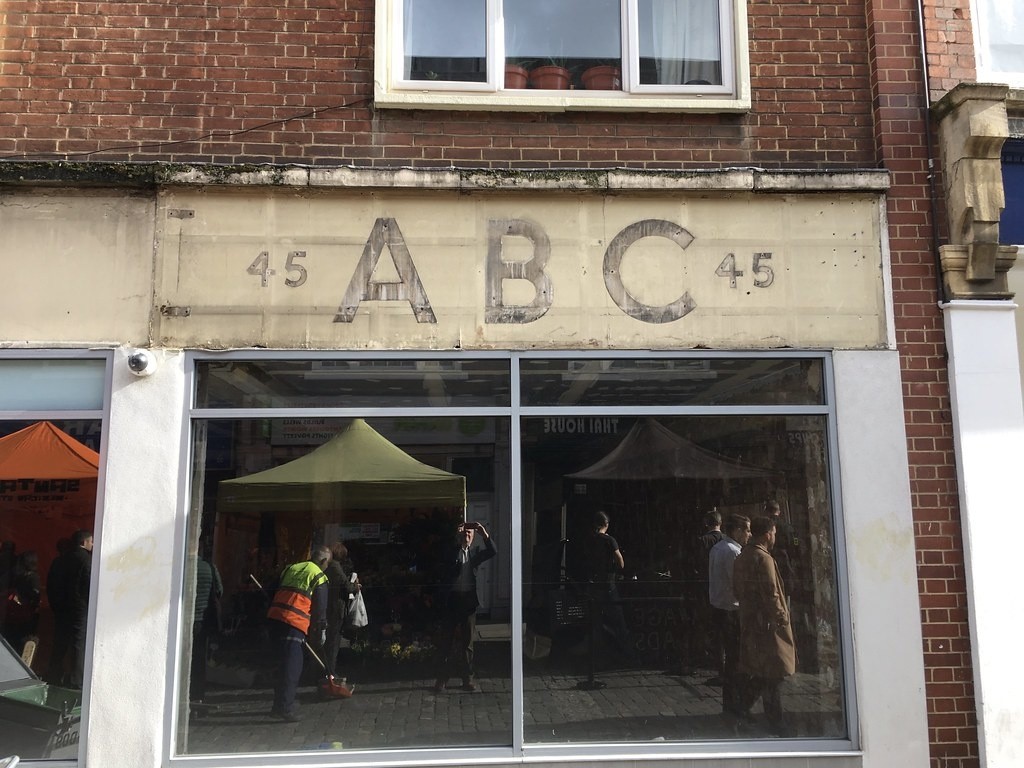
[583,509,642,670]
[436,521,500,694]
[692,498,813,739]
[190,539,224,719]
[0,528,93,690]
[246,541,362,723]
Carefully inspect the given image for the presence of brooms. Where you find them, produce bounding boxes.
[250,574,346,687]
[321,646,352,697]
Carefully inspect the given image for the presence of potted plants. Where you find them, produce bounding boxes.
[505,25,621,90]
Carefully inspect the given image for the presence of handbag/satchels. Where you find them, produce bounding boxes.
[348,590,368,630]
[202,562,225,632]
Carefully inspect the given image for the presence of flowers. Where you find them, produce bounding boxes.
[347,629,438,665]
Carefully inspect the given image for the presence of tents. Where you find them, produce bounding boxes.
[211,419,470,570]
[560,416,791,592]
[1,421,101,575]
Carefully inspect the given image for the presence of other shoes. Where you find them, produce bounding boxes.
[434,680,445,692]
[462,681,475,695]
